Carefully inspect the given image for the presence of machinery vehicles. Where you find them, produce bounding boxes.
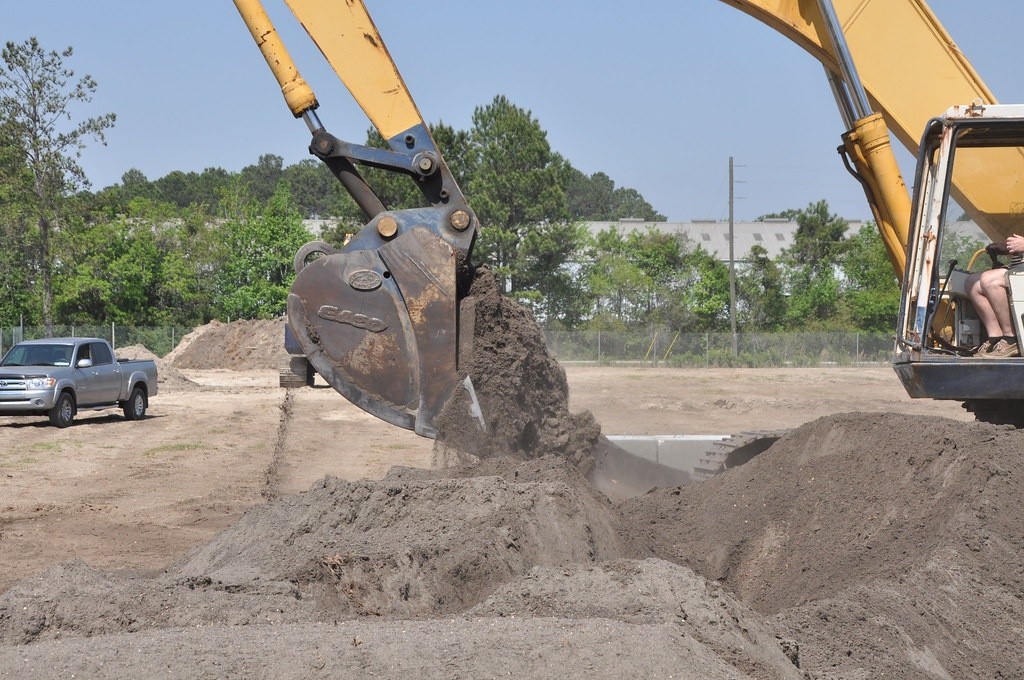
[234,0,1023,480]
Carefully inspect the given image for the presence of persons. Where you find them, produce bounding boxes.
[970,234,1024,357]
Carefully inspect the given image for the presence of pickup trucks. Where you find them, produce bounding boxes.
[0,338,158,428]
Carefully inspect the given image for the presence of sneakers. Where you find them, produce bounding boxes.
[973,341,990,357]
[983,339,1018,358]
